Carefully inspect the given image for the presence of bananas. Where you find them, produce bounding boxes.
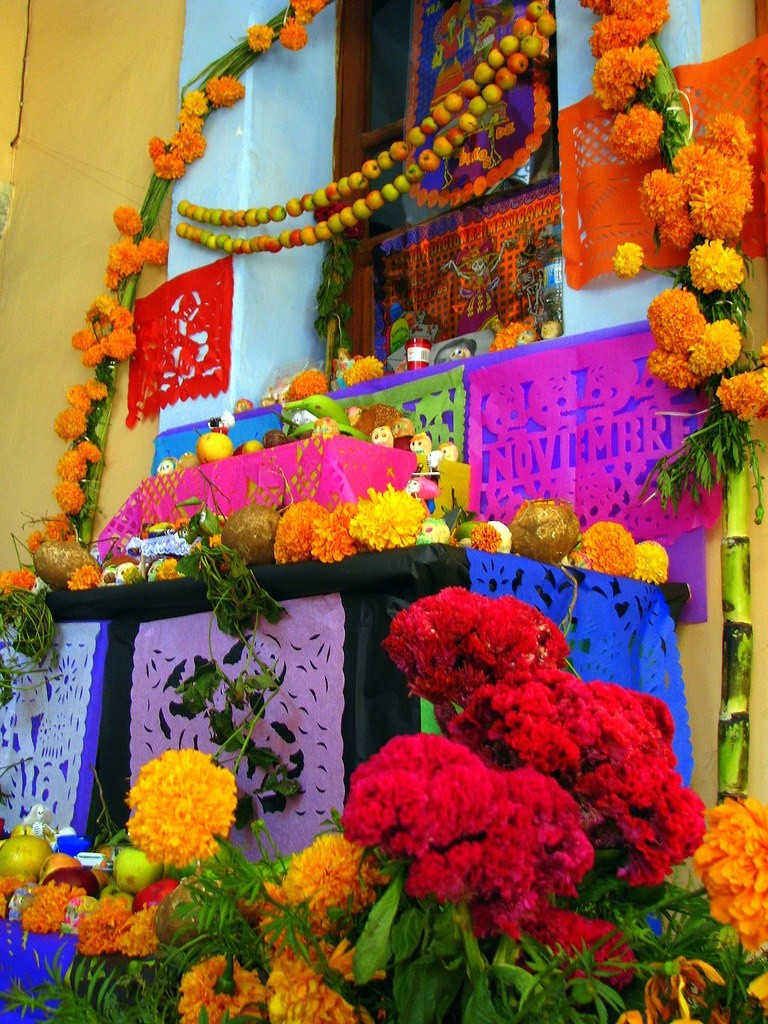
[284,394,351,426]
[288,422,371,443]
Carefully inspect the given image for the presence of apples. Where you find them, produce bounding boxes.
[242,439,263,454]
[0,824,180,915]
[196,432,233,464]
[455,520,481,539]
[176,1,556,255]
[234,399,253,413]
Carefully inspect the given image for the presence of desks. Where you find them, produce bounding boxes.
[0,543,693,864]
